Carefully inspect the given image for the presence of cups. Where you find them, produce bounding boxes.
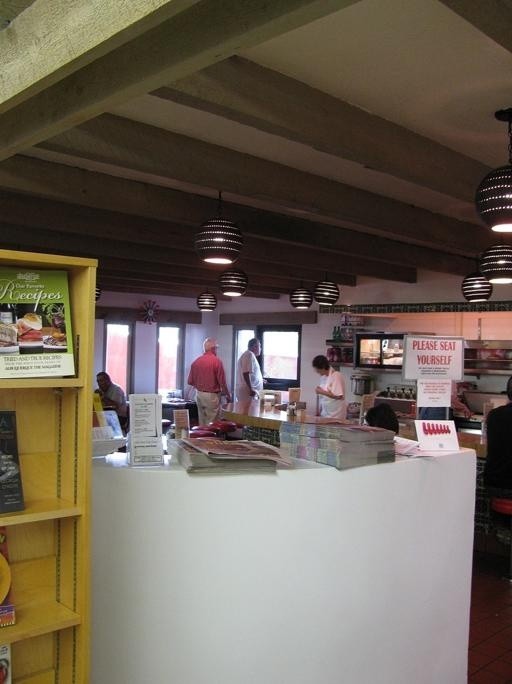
[286,401,297,416]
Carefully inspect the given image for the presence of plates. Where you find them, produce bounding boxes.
[18,341,43,348]
[0,346,20,352]
[43,335,67,349]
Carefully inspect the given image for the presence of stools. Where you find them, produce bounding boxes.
[489,500,512,578]
[460,273,491,303]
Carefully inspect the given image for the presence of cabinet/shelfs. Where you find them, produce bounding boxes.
[325,340,354,365]
[0,250,98,683]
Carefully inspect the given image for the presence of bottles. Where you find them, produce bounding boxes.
[393,343,399,352]
[289,388,301,403]
[0,303,16,325]
[275,392,281,404]
[333,327,336,338]
[410,402,416,415]
[264,392,276,414]
[336,326,341,337]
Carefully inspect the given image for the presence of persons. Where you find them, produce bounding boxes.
[235,338,264,418]
[312,355,347,422]
[483,377,512,542]
[94,372,129,453]
[419,381,471,422]
[187,337,231,427]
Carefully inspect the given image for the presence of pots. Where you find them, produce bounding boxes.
[350,375,377,395]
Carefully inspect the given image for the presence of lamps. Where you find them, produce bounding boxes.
[194,219,244,265]
[477,167,512,233]
[197,292,216,311]
[315,281,340,305]
[289,288,314,310]
[480,245,512,285]
[217,269,248,296]
